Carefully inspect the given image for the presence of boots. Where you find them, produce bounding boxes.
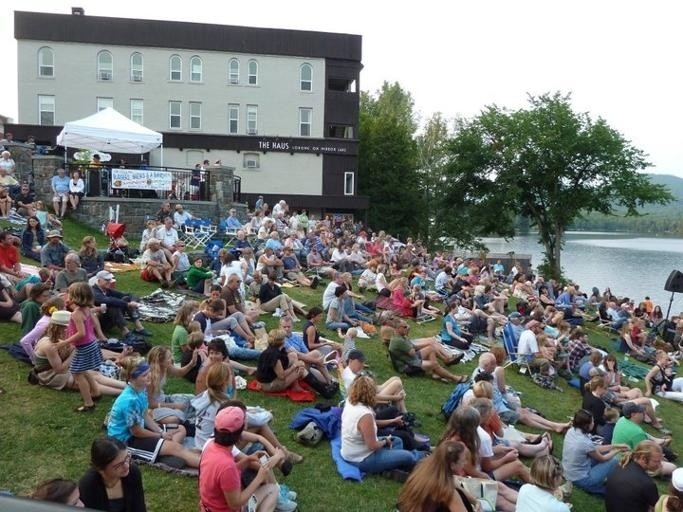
[305,374,340,401]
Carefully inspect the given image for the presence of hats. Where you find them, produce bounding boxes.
[672,468,683,492]
[97,270,114,281]
[47,230,64,239]
[51,311,71,326]
[349,349,367,361]
[214,407,245,433]
[622,403,647,414]
[510,312,524,321]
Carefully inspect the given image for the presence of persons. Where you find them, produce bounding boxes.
[76,436,147,512]
[196,401,297,511]
[560,389,683,512]
[20,295,134,367]
[144,346,199,426]
[189,159,223,200]
[190,363,305,466]
[0,187,15,218]
[473,380,554,460]
[0,280,23,323]
[0,151,15,172]
[435,405,518,512]
[341,377,434,484]
[589,287,683,387]
[397,440,481,512]
[0,268,51,301]
[367,232,507,381]
[55,253,88,292]
[0,231,24,277]
[32,475,85,507]
[90,270,154,340]
[87,273,116,289]
[22,217,46,261]
[31,310,127,396]
[467,398,572,499]
[253,194,366,398]
[507,263,589,387]
[515,454,572,512]
[107,358,201,469]
[41,230,70,270]
[26,135,35,145]
[52,280,105,413]
[0,167,17,187]
[21,284,49,337]
[105,222,135,263]
[34,200,61,237]
[79,235,104,277]
[55,290,108,341]
[120,158,129,169]
[14,184,36,217]
[0,133,14,144]
[89,154,102,170]
[137,202,254,376]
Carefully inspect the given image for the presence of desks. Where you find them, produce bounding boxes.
[183,225,217,250]
[224,226,259,250]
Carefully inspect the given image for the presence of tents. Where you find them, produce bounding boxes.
[56,107,163,197]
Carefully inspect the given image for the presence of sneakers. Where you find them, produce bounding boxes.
[157,455,187,471]
[133,326,153,335]
[284,449,303,464]
[281,489,297,500]
[127,332,136,340]
[275,499,298,512]
[445,353,465,368]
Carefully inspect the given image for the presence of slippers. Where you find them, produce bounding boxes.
[549,440,553,454]
[541,430,551,442]
[431,377,448,384]
[456,374,470,384]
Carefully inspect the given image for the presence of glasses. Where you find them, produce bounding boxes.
[236,279,242,284]
[23,188,28,191]
[401,326,408,330]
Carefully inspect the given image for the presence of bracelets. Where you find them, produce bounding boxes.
[158,402,160,407]
